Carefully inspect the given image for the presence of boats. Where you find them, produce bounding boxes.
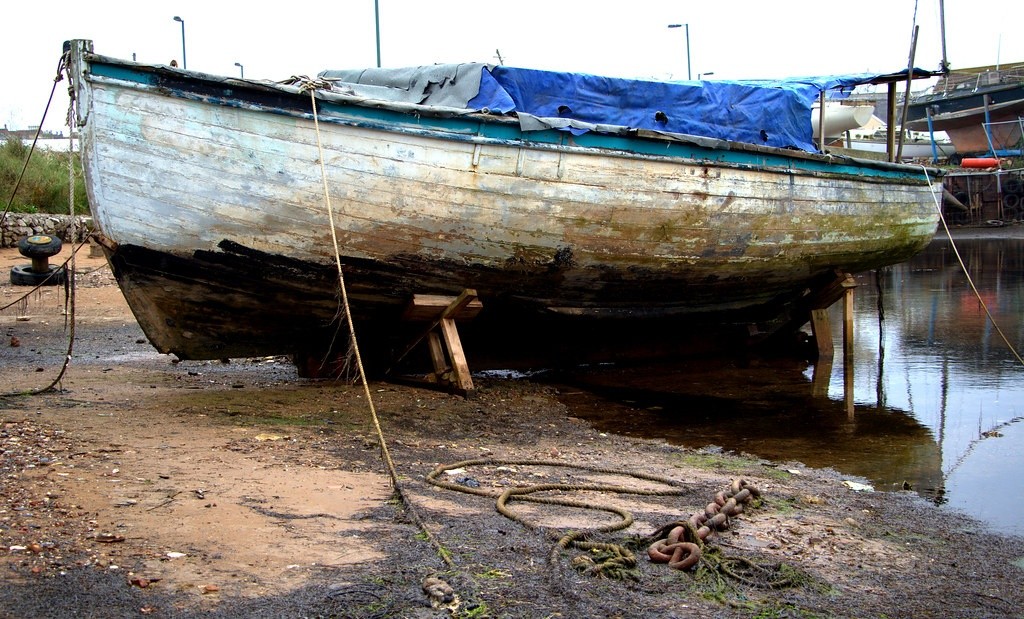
[896,61,1024,154]
[63,38,949,360]
[841,129,956,157]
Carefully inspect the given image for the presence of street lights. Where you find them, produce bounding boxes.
[669,24,691,81]
[235,62,243,79]
[698,72,714,81]
[173,16,186,69]
